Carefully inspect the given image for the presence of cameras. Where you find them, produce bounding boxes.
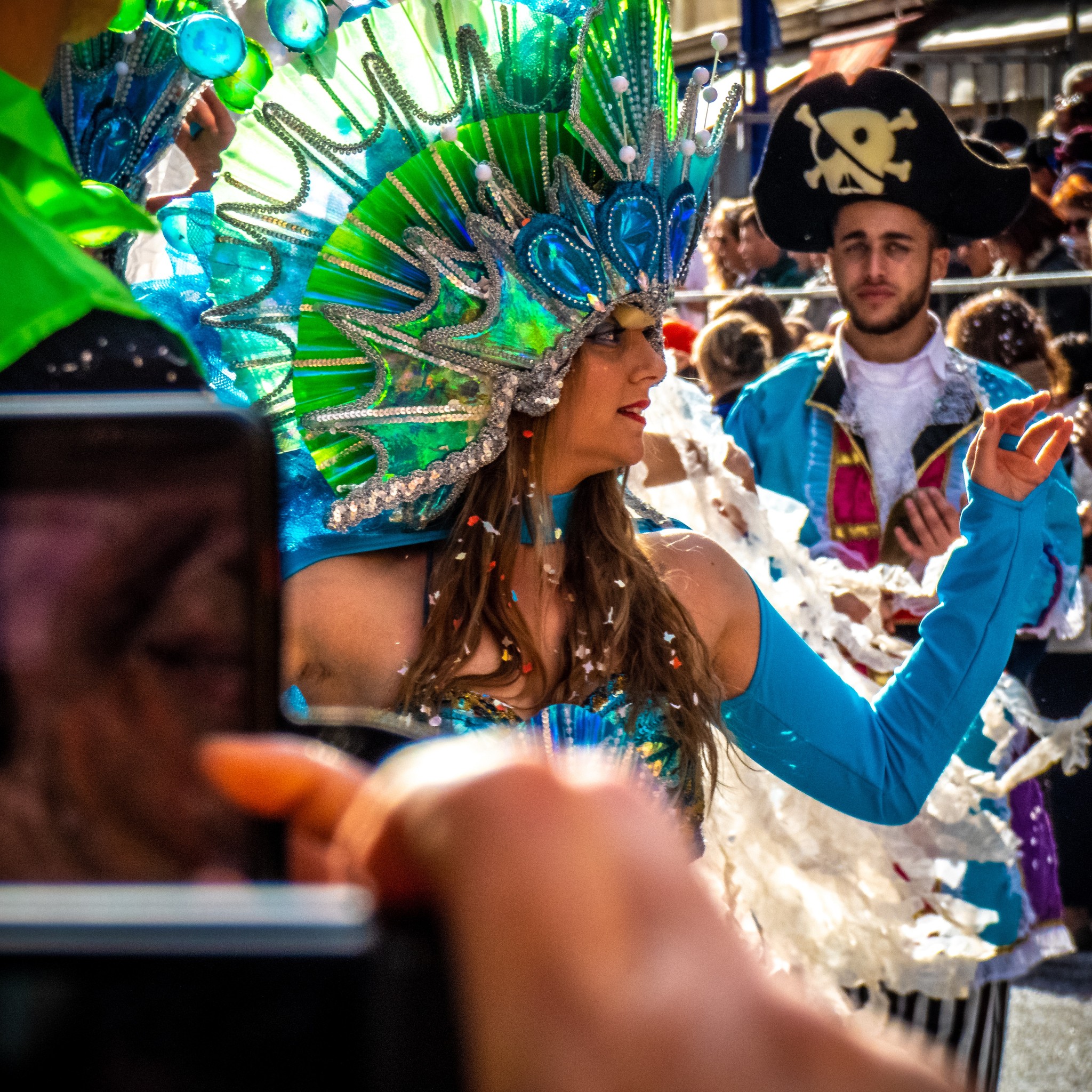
[0,879,462,1092]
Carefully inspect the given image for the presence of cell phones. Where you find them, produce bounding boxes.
[0,390,289,883]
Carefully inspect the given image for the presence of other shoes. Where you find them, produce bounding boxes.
[1059,906,1092,951]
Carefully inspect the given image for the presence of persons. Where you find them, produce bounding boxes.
[190,722,982,1092]
[660,59,1092,940]
[686,66,1083,1092]
[277,294,1076,866]
[37,530,250,890]
[0,0,237,395]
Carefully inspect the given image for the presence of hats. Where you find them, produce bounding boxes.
[748,65,1032,250]
[980,116,1029,150]
[1053,123,1092,163]
